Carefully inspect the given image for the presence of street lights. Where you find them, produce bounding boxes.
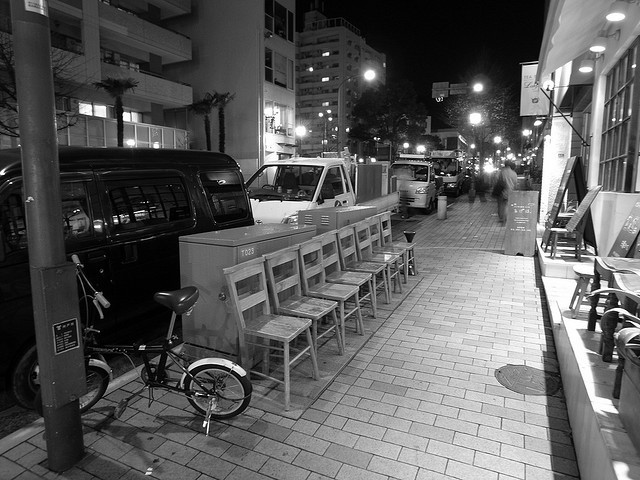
[336,69,375,158]
[295,126,306,158]
[374,137,381,156]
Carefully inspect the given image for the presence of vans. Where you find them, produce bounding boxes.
[0,146,255,407]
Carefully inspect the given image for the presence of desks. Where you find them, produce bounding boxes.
[588,256,640,354]
[602,272,640,399]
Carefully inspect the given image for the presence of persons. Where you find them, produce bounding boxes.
[491,160,518,227]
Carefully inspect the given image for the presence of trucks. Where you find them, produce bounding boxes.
[245,157,400,224]
[388,160,445,215]
[429,150,469,198]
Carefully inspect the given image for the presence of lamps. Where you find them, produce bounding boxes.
[579,54,604,73]
[589,29,620,53]
[605,0,639,23]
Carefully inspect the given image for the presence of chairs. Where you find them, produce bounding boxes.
[551,207,590,261]
[354,219,403,300]
[222,257,319,411]
[337,224,390,308]
[312,229,377,332]
[298,239,365,346]
[380,211,417,278]
[262,245,344,379]
[365,215,407,293]
[569,244,640,319]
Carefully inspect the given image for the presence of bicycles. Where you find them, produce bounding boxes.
[12,254,252,438]
